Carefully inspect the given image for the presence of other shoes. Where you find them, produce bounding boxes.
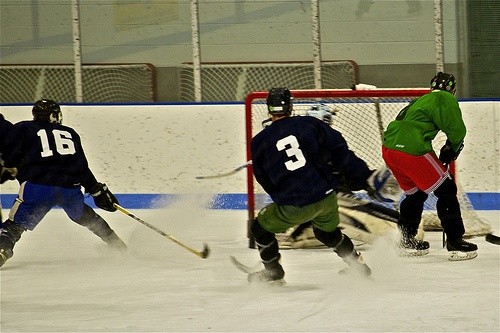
[102,230,128,250]
[0,246,14,268]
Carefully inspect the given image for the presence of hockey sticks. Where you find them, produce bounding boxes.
[230,220,312,274]
[112,202,209,259]
[442,228,447,248]
[195,159,252,180]
[485,233,500,245]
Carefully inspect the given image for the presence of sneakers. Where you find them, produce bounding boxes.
[338,252,372,277]
[446,237,478,261]
[248,265,287,287]
[395,238,430,257]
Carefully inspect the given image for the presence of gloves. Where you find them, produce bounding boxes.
[90,182,119,212]
[439,138,465,163]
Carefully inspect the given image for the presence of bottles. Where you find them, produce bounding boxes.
[350,84,378,90]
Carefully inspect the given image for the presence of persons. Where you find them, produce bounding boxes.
[0,100,128,267]
[246,87,371,285]
[382,72,478,252]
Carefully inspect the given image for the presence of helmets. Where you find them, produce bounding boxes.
[307,104,332,124]
[32,98,63,123]
[266,87,293,116]
[430,71,456,97]
